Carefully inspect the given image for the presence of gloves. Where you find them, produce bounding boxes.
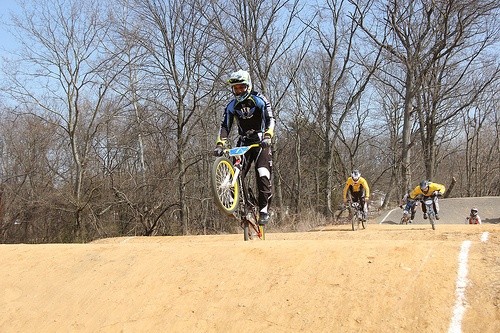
[260,139,268,147]
[213,146,223,157]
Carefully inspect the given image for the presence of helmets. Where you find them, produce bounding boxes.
[420,180,429,194]
[351,169,360,184]
[470,207,478,217]
[230,69,252,102]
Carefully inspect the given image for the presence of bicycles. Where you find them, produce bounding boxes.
[399,198,419,225]
[211,134,268,242]
[347,199,368,231]
[413,193,443,230]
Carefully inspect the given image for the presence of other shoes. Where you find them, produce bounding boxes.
[435,214,440,220]
[409,220,412,223]
[258,213,269,224]
[423,213,428,219]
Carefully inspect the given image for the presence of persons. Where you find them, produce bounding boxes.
[399,190,417,223]
[409,181,446,219]
[213,70,275,225]
[465,208,481,225]
[343,169,370,220]
